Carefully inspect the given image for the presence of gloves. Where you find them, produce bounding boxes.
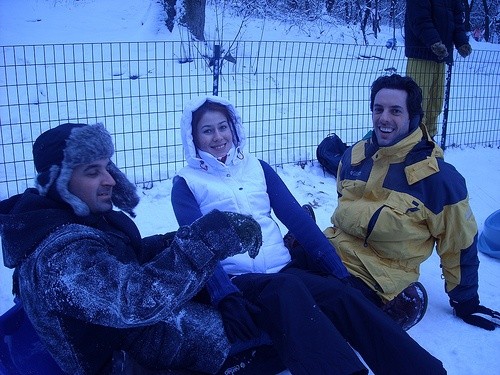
[456,43,472,57]
[224,211,262,259]
[460,305,499,331]
[218,291,264,343]
[432,42,447,58]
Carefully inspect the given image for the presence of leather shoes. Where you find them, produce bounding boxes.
[385,281,428,332]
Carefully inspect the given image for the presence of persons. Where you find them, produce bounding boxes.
[171,95,447,375]
[0,122,428,375]
[321,74,500,331]
[404,0,473,137]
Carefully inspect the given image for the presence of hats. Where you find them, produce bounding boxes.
[478,209,500,258]
[32,122,141,219]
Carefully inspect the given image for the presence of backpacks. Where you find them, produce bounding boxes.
[316,132,352,178]
